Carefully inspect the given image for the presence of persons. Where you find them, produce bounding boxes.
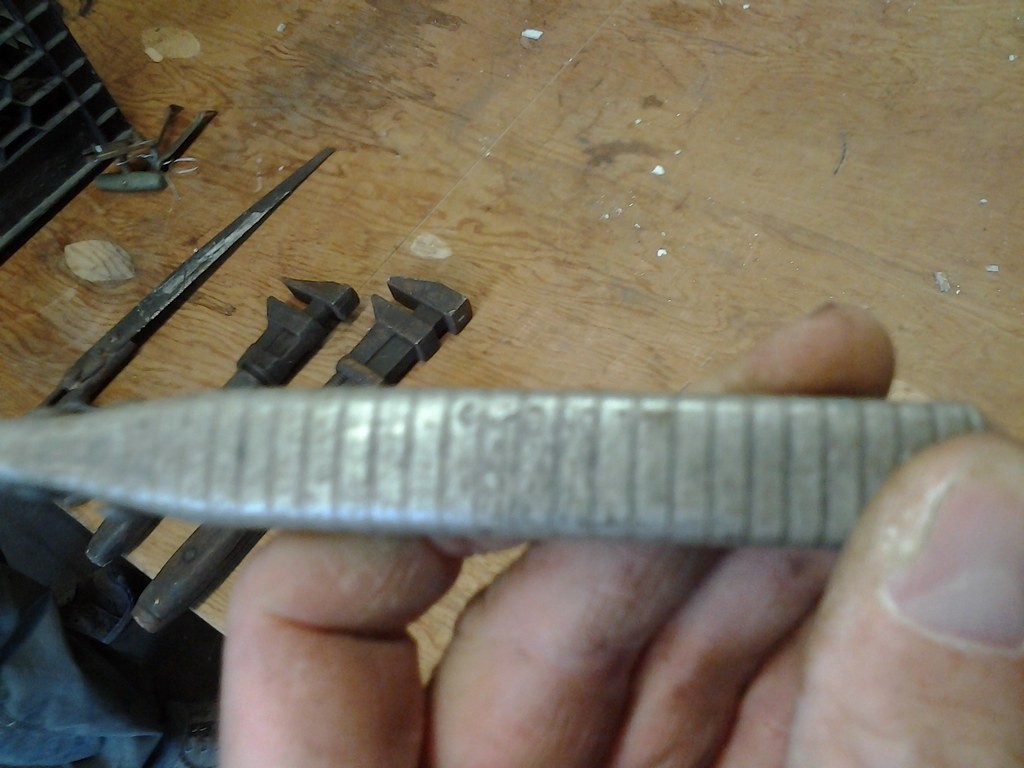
[1,473,216,768]
[216,294,1023,768]
